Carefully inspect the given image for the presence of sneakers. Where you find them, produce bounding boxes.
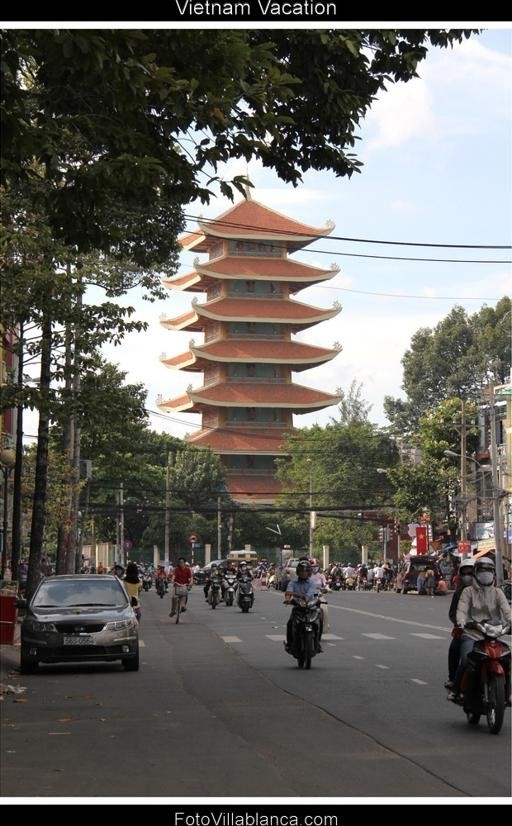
[169,607,188,617]
[444,677,457,703]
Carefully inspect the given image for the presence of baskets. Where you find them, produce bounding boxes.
[175,586,187,596]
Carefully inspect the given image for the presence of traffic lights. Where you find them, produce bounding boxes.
[378,527,394,544]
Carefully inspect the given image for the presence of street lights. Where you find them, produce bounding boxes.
[443,450,487,516]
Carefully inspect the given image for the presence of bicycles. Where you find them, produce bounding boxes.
[172,582,191,624]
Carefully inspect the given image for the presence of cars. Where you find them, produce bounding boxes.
[13,573,140,674]
[394,555,445,594]
[274,558,301,591]
[193,559,231,585]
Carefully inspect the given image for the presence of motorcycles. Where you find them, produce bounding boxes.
[282,589,327,670]
[448,621,512,735]
[143,575,169,599]
[324,570,395,593]
[207,575,255,613]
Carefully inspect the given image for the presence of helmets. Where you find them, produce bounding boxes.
[296,557,320,578]
[335,563,382,568]
[204,559,276,571]
[458,557,495,570]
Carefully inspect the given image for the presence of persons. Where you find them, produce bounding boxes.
[78,554,447,650]
[446,557,512,706]
[444,559,475,689]
[39,559,51,577]
[21,557,28,587]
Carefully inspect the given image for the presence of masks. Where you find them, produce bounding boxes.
[476,572,493,584]
[461,576,474,586]
